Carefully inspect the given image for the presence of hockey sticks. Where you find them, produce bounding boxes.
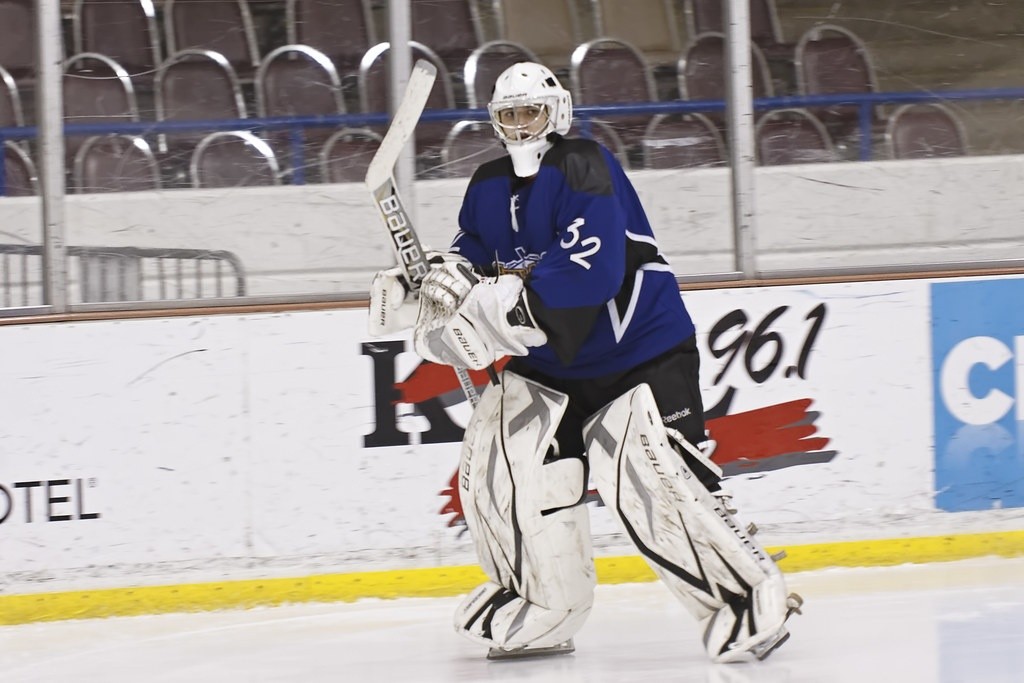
[364,58,482,410]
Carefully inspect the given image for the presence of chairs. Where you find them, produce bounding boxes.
[0,1,972,196]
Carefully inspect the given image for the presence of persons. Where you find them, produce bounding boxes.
[369,62,801,660]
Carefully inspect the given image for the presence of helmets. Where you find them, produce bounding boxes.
[487,62,573,178]
